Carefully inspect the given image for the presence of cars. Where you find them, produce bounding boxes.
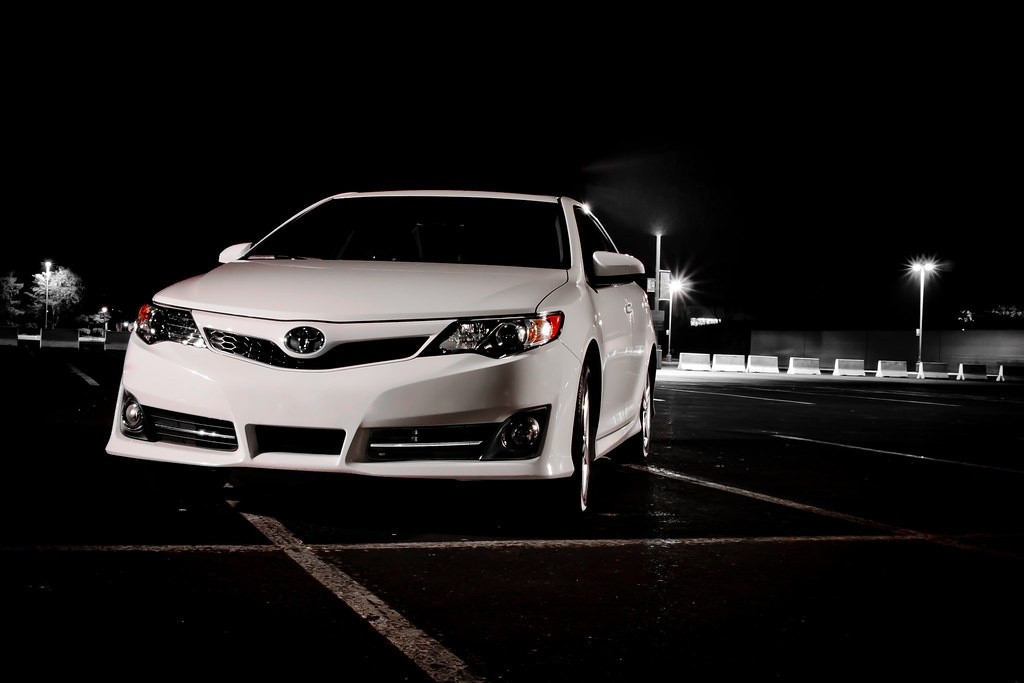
[103,189,658,525]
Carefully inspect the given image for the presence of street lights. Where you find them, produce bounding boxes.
[912,262,936,373]
[665,279,681,361]
